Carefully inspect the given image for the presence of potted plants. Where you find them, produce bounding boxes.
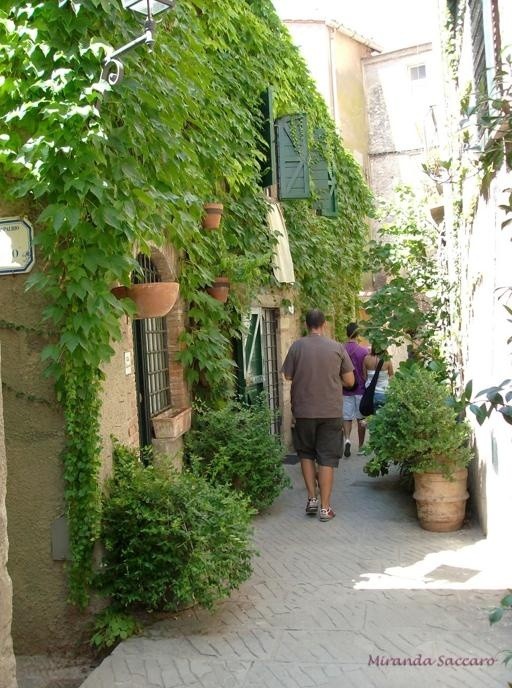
[363,361,472,531]
[202,202,230,304]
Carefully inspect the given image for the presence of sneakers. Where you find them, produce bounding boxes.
[306,496,319,512]
[344,439,351,457]
[356,450,365,455]
[320,505,336,522]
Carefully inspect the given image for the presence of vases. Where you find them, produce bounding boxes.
[151,406,192,440]
[108,280,179,321]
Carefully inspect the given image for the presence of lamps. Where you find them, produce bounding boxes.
[83,0,176,115]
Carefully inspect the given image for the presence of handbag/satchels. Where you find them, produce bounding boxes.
[359,390,374,416]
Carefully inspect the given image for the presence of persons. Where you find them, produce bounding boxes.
[282,309,356,522]
[341,323,369,457]
[363,338,395,416]
